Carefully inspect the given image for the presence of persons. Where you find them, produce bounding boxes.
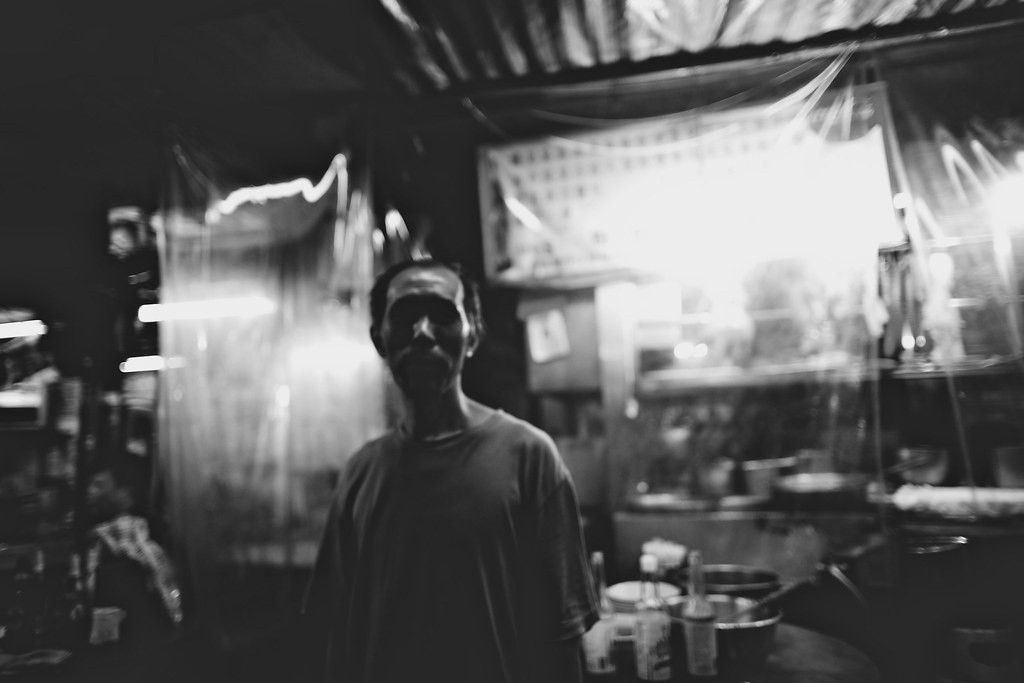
[86,455,181,625]
[302,258,602,683]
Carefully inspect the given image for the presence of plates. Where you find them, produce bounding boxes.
[605,581,683,613]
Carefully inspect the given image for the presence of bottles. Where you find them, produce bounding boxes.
[681,549,720,683]
[579,552,618,683]
[632,554,672,683]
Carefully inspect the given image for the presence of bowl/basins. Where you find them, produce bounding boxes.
[674,563,782,599]
[664,594,783,672]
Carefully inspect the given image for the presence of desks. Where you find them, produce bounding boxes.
[757,624,880,683]
